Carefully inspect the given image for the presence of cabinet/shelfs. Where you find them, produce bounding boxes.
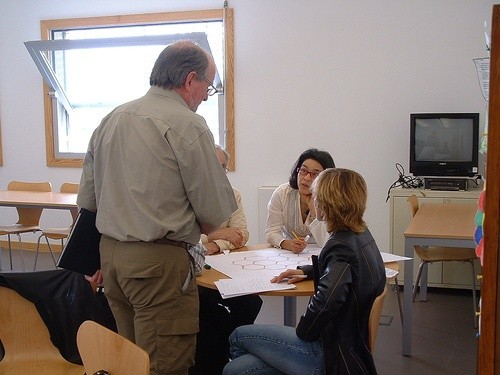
[389,188,484,290]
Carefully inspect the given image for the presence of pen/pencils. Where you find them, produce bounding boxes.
[273,275,308,279]
[291,231,303,247]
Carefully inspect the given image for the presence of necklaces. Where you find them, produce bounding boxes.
[301,199,309,215]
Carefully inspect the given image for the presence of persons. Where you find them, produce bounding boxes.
[190,145,385,375]
[69,40,237,375]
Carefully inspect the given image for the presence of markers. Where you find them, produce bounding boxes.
[203,263,211,269]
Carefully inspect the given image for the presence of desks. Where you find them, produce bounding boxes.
[195,244,399,357]
[0,190,79,210]
[401,200,479,358]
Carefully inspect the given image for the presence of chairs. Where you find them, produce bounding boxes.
[75,319,150,375]
[0,269,96,375]
[406,194,479,329]
[33,183,81,271]
[368,286,387,352]
[0,181,52,271]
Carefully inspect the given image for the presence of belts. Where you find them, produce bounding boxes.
[154,238,187,249]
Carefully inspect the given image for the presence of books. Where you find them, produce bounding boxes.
[56,207,102,277]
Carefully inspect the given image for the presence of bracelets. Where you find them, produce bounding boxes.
[310,214,316,220]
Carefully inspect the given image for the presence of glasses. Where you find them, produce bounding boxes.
[223,167,230,174]
[297,168,319,179]
[203,76,218,96]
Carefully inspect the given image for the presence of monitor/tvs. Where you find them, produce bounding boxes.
[409,113,479,177]
